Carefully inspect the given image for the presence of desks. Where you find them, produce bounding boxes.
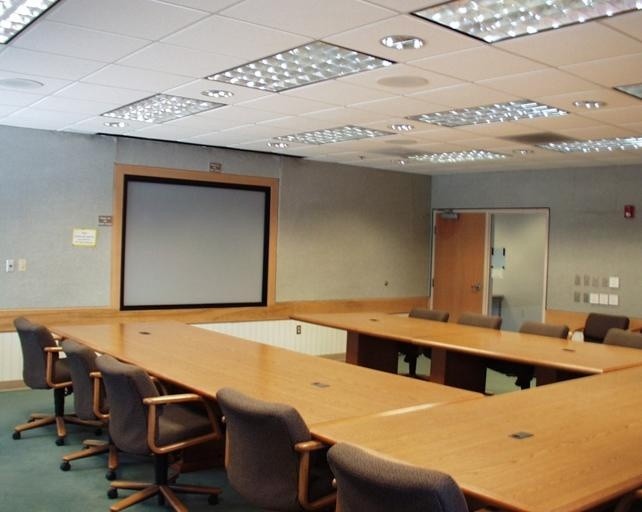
[306,366,641,511]
[289,311,642,372]
[43,324,489,429]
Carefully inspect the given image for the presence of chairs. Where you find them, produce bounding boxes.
[328,441,467,510]
[405,305,642,391]
[213,386,333,510]
[94,352,223,512]
[59,333,152,480]
[14,316,101,446]
